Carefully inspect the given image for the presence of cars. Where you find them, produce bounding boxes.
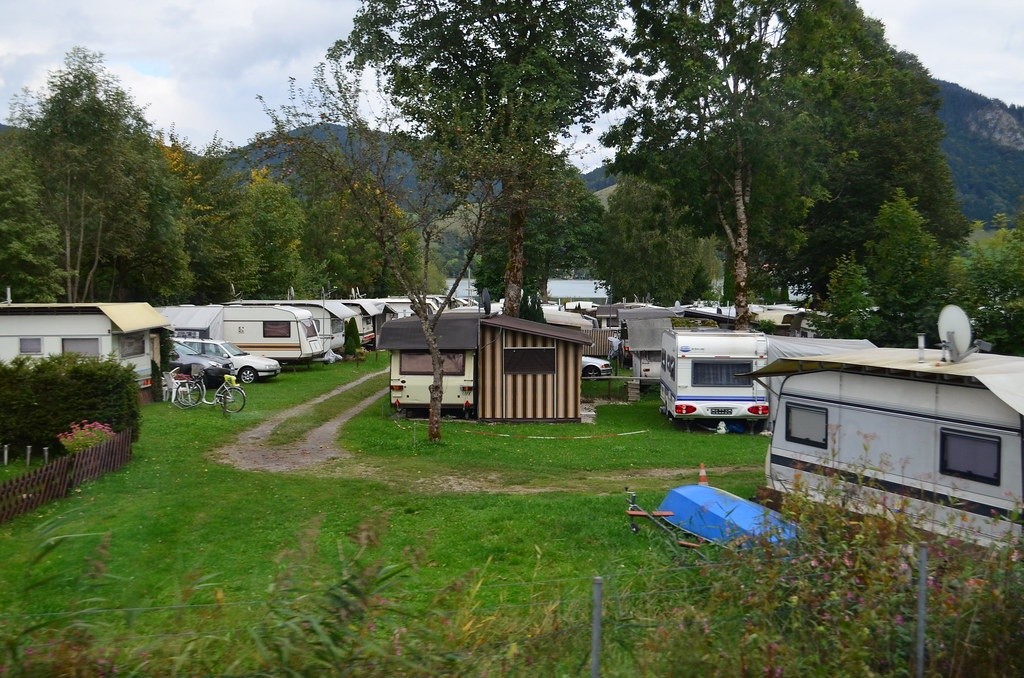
[167,338,236,393]
[582,355,613,382]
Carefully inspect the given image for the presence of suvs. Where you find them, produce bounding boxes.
[171,336,282,385]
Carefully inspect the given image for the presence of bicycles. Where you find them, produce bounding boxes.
[175,368,247,414]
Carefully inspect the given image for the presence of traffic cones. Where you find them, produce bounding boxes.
[698,463,709,487]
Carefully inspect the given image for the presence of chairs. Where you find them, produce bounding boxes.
[162,367,194,404]
